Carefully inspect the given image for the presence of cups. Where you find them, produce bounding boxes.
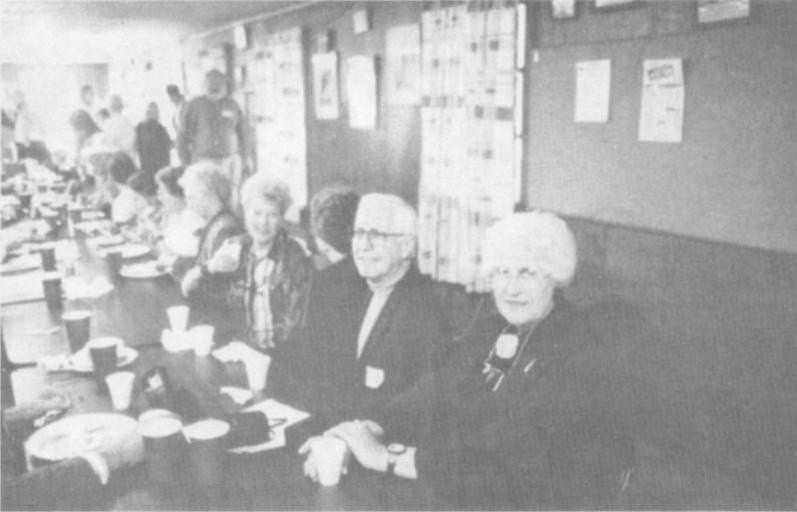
[37,207,233,485]
[239,354,272,391]
[312,436,346,488]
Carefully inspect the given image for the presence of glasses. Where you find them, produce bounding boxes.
[351,231,403,245]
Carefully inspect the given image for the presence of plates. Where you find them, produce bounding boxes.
[3,157,173,303]
[26,412,144,461]
[68,340,138,370]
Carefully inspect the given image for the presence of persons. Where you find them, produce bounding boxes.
[303,187,367,388]
[0,71,314,358]
[322,193,455,427]
[0,387,110,512]
[299,211,623,512]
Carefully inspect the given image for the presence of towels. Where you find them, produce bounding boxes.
[225,397,314,455]
[35,400,69,428]
[160,328,214,354]
[212,340,271,391]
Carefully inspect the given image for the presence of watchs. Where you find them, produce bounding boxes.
[386,442,406,476]
